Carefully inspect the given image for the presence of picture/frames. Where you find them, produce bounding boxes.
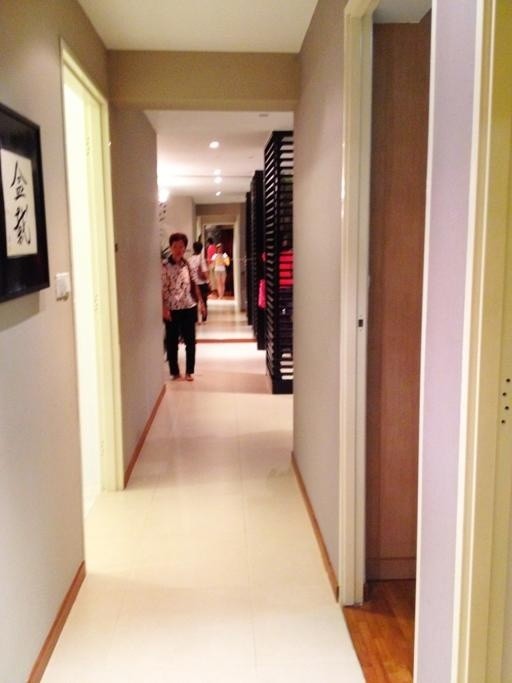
[0,103,51,304]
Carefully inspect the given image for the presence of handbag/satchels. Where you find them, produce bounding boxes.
[184,258,199,302]
[196,254,208,280]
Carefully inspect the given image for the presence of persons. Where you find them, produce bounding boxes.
[185,239,211,327]
[209,241,232,301]
[203,236,219,297]
[158,230,207,382]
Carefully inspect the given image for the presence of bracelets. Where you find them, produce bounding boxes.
[200,300,205,304]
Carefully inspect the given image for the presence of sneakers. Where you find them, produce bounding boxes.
[170,373,194,382]
